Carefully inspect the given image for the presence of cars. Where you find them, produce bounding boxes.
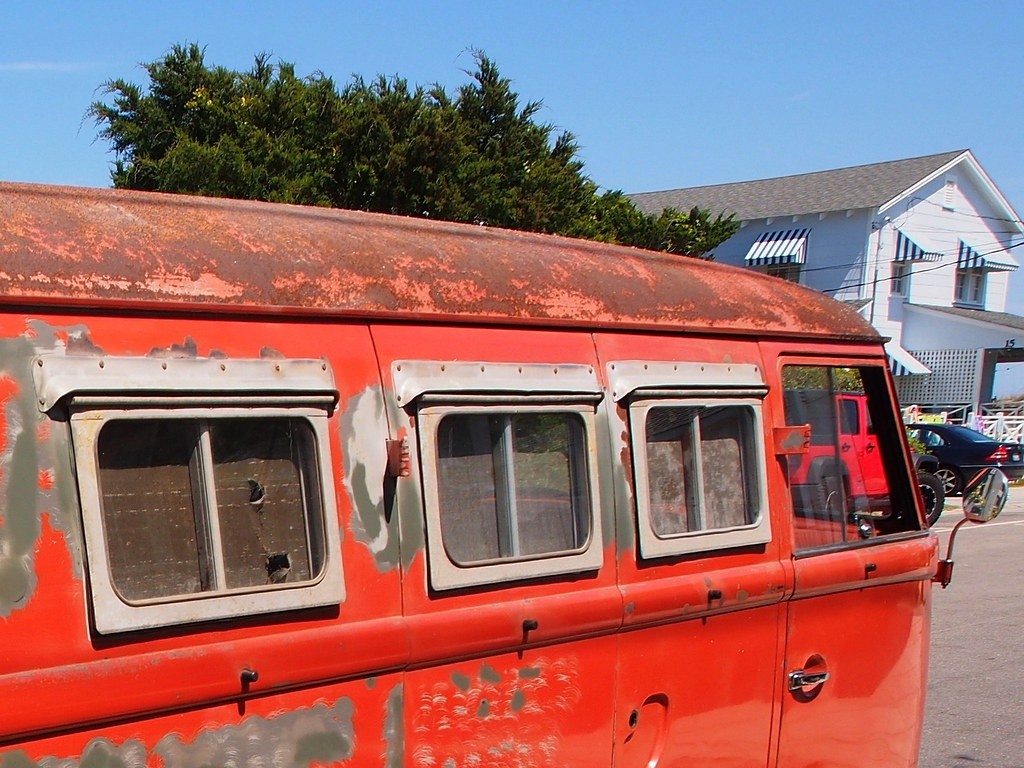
[904,423,1024,499]
